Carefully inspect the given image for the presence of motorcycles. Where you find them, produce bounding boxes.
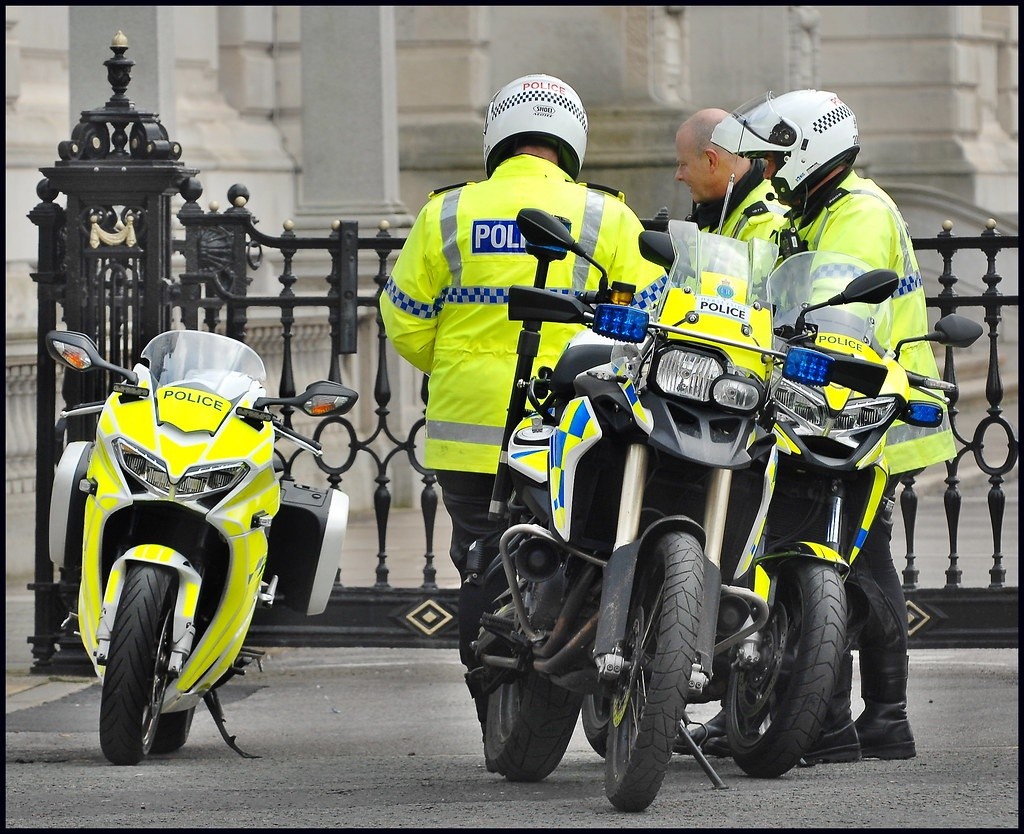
[26,331,362,769]
[450,208,982,812]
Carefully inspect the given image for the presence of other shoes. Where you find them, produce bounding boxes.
[672,709,730,757]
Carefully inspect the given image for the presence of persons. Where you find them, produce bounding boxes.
[672,89,957,763]
[379,70,668,674]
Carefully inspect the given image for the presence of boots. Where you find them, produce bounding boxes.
[797,654,861,764]
[854,649,916,759]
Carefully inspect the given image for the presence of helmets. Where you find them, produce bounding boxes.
[483,72,589,181]
[710,90,860,205]
